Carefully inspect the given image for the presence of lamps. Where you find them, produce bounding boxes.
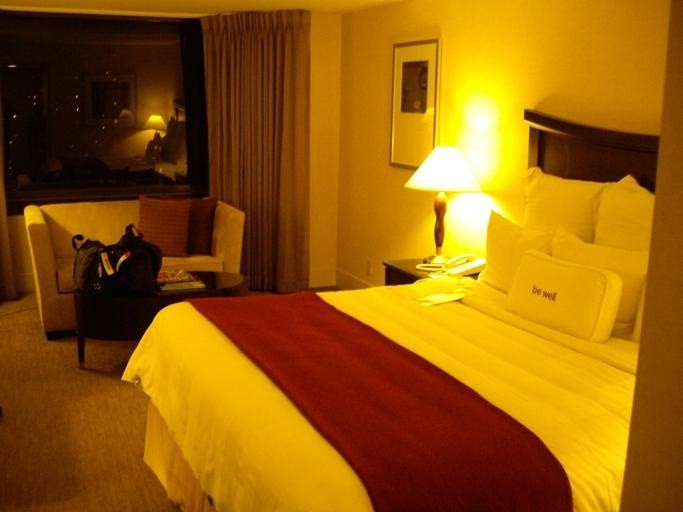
[142,114,168,141]
[402,146,480,263]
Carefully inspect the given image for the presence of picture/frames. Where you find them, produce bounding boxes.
[388,38,439,169]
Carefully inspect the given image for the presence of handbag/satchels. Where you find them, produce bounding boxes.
[69,223,164,298]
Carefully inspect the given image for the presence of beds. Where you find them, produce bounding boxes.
[126,110,656,512]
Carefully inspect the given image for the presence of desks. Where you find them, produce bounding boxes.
[68,271,244,368]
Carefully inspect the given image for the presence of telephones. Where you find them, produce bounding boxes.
[442,254,487,276]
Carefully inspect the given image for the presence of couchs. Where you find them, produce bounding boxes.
[20,193,246,338]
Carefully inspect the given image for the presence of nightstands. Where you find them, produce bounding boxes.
[378,254,479,285]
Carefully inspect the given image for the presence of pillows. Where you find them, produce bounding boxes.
[133,194,191,255]
[481,163,652,347]
[150,196,215,255]
